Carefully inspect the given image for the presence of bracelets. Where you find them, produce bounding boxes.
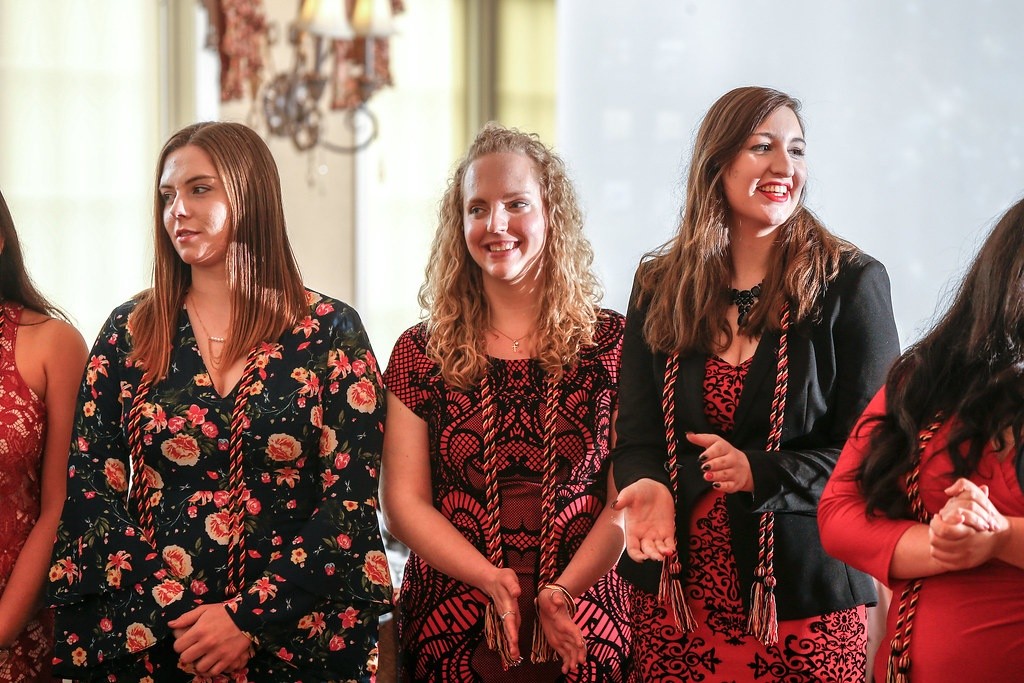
[539,584,577,621]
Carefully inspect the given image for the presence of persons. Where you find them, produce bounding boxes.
[381,127,642,683]
[42,121,399,683]
[816,199,1023,683]
[0,188,87,683]
[611,88,902,683]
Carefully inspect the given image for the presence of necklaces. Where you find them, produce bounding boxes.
[490,324,529,353]
[726,279,766,328]
[188,290,228,370]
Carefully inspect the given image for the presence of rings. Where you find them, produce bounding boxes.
[502,610,516,622]
